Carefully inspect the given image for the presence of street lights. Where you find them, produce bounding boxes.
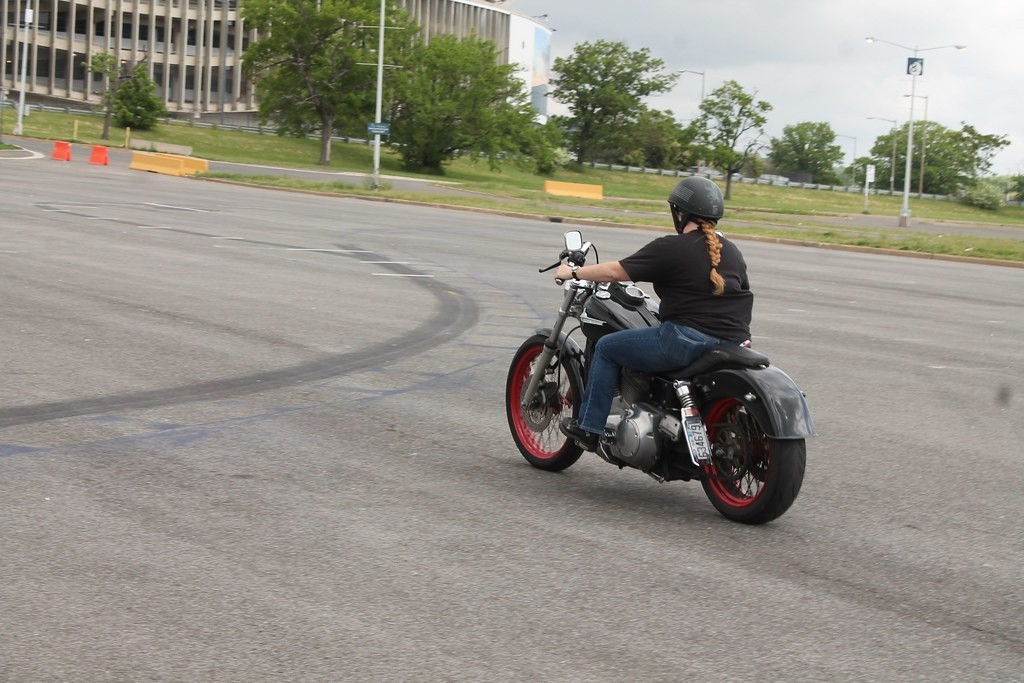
[678,69,704,121]
[902,94,928,198]
[835,135,855,190]
[867,117,898,195]
[866,36,967,226]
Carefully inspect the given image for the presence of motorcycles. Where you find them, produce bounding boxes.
[505,228,814,528]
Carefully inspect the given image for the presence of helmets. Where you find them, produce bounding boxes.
[668,176,724,218]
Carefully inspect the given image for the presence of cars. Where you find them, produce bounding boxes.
[569,152,578,162]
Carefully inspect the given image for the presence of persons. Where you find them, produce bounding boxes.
[554,176,753,453]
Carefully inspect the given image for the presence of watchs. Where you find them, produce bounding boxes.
[571,266,582,281]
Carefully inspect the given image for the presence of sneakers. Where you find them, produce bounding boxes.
[559,416,600,452]
